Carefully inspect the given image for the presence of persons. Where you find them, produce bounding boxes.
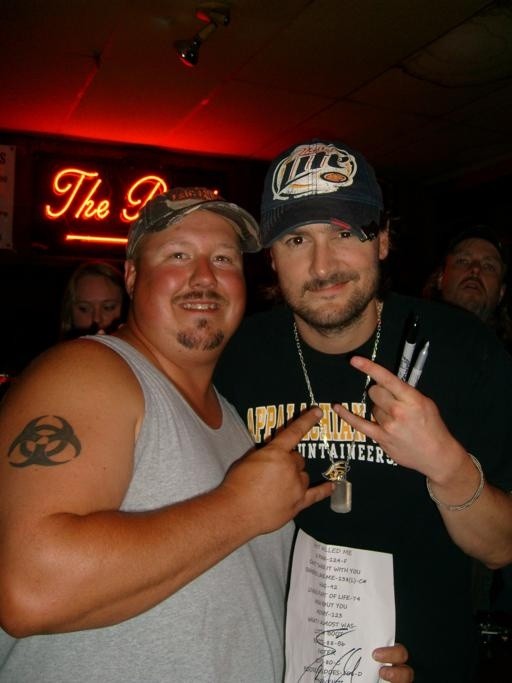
[55,258,133,338]
[206,134,511,683]
[435,233,506,322]
[0,181,419,682]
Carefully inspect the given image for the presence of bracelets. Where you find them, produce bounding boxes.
[425,449,484,512]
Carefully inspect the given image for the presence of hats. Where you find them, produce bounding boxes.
[257,139,390,249]
[124,186,264,257]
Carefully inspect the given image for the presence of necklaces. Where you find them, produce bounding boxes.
[287,289,383,517]
